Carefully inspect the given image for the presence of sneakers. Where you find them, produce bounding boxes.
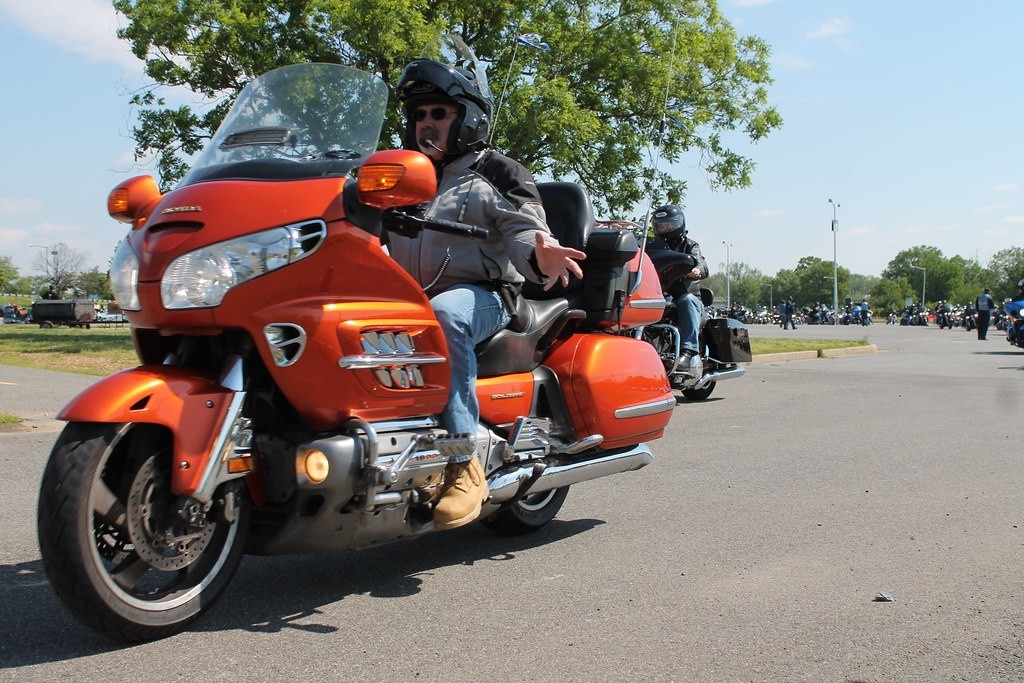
[662,350,691,371]
[433,448,490,530]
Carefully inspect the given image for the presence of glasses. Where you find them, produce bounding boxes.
[410,107,460,122]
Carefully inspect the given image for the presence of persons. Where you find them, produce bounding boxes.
[993,298,1012,325]
[886,300,976,327]
[779,300,786,328]
[644,205,709,369]
[731,299,874,327]
[783,295,798,330]
[42,284,61,300]
[1007,279,1024,345]
[346,58,587,531]
[976,288,999,341]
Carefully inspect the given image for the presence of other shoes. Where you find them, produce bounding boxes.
[784,328,789,330]
[780,323,782,327]
[793,326,798,330]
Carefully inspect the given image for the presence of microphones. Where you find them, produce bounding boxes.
[424,140,471,155]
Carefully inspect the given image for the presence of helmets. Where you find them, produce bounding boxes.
[912,304,915,306]
[943,300,946,303]
[393,34,493,164]
[937,300,942,304]
[904,306,907,308]
[1018,279,1024,294]
[999,302,1005,306]
[652,205,688,249]
[732,296,866,309]
[891,307,894,309]
[918,303,921,305]
[956,304,960,307]
[968,301,972,305]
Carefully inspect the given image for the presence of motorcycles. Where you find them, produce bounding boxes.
[718,301,1024,352]
[622,247,753,403]
[34,62,681,640]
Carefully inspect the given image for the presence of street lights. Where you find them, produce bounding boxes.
[28,242,64,278]
[828,198,841,322]
[910,266,926,306]
[759,283,773,310]
[722,240,733,311]
[823,276,838,316]
